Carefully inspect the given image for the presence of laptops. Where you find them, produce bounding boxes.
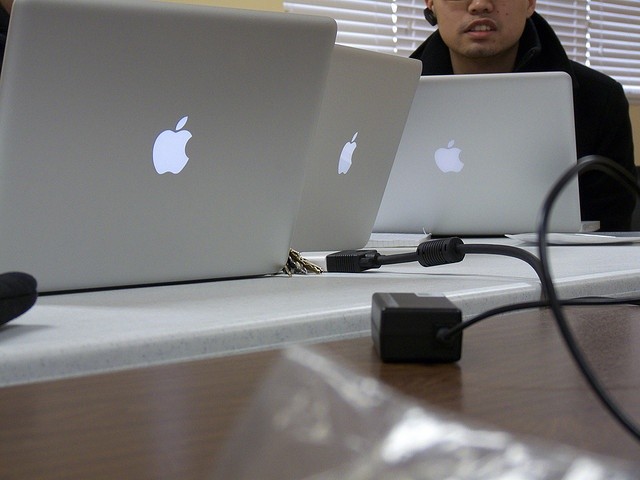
[1,0,338,295]
[372,71,601,234]
[290,44,424,252]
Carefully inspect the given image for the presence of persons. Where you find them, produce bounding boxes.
[406,0,635,231]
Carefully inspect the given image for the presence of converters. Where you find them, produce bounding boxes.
[371,293,462,364]
